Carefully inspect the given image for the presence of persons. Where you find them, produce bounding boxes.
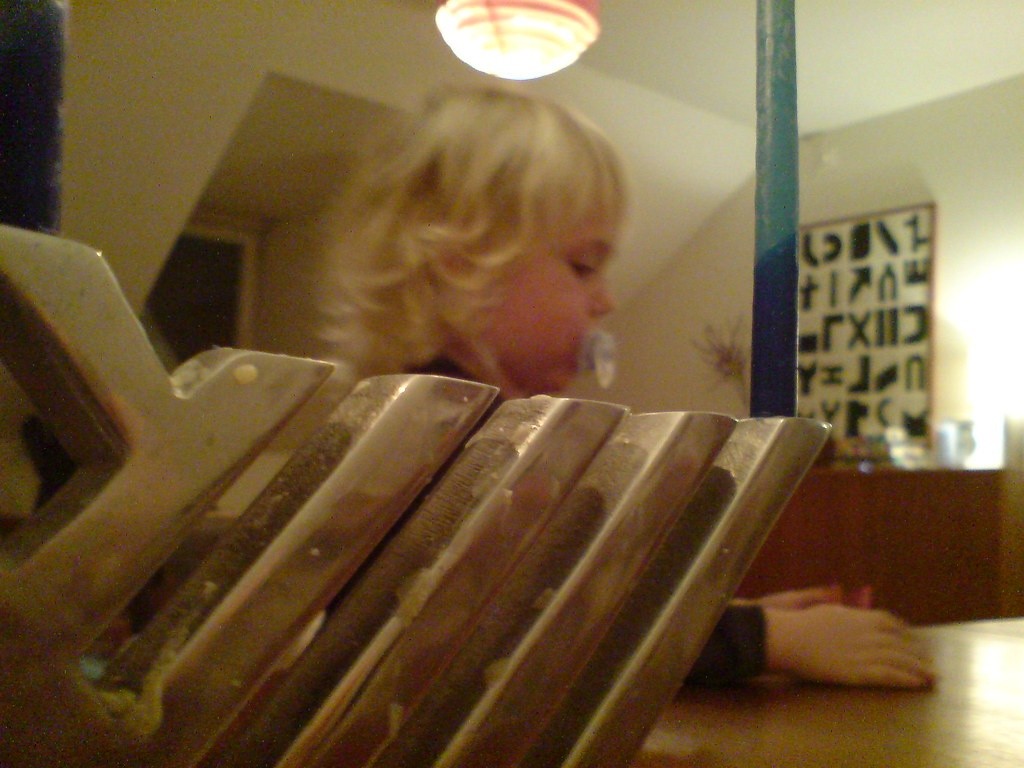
[257,87,934,692]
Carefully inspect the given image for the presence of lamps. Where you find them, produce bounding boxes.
[434,0,602,80]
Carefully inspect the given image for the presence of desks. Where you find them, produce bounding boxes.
[633,616,1024,768]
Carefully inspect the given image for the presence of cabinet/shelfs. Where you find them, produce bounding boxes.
[735,468,1024,624]
